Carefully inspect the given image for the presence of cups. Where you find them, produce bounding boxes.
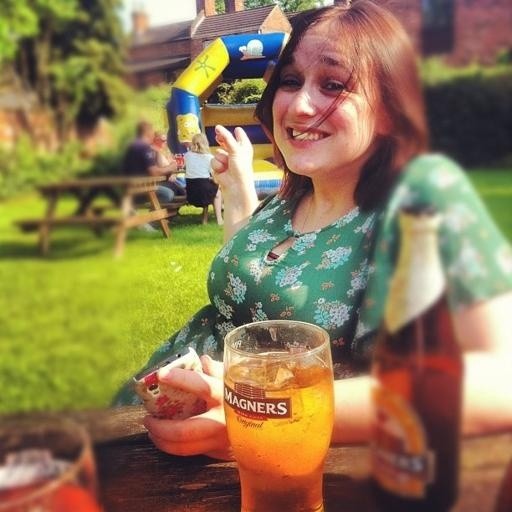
[222,317,337,510]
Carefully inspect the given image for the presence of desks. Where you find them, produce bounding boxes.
[1,381,511,511]
[32,173,172,257]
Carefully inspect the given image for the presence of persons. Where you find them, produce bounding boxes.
[151,129,185,195]
[184,131,225,227]
[107,1,512,467]
[122,117,179,236]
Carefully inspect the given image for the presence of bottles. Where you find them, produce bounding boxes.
[0,411,107,510]
[369,207,464,509]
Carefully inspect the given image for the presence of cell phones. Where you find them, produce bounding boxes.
[133,347,207,421]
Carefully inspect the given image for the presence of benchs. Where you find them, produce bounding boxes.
[14,196,189,231]
